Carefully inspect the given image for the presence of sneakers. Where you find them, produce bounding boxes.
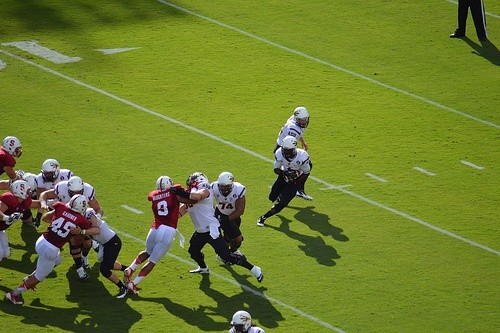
[123,268,131,284]
[84,260,89,268]
[23,276,36,289]
[76,268,89,279]
[256,216,265,226]
[127,282,139,294]
[296,191,313,200]
[6,292,23,305]
[189,266,209,273]
[251,265,263,282]
[116,285,128,298]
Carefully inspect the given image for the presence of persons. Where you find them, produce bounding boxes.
[212,172,247,264]
[0,136,129,303]
[170,172,263,282]
[123,176,198,292]
[257,136,310,227]
[450,0,488,42]
[229,311,264,333]
[274,107,313,200]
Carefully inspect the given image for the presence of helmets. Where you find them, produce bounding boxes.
[218,171,233,195]
[187,172,208,189]
[293,106,309,127]
[3,135,22,158]
[230,310,251,332]
[156,176,174,190]
[282,135,297,157]
[10,158,88,215]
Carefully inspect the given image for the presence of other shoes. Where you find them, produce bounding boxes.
[450,33,465,38]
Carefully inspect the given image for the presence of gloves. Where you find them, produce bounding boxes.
[282,170,298,182]
[218,214,229,223]
[9,212,23,222]
[34,212,42,227]
[171,187,185,196]
